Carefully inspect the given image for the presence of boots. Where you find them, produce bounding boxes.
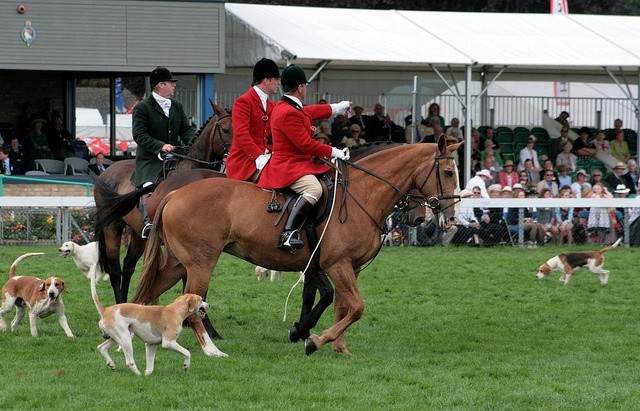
[278,195,314,251]
[138,194,152,238]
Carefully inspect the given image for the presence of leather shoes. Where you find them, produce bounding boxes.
[474,241,480,247]
[466,239,472,246]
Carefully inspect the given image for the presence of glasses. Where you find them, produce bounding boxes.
[519,174,526,177]
[560,129,569,133]
[474,192,480,194]
[545,173,553,176]
[352,131,359,133]
[593,174,601,176]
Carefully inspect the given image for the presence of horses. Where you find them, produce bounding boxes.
[132,133,465,356]
[87,140,413,342]
[93,97,233,304]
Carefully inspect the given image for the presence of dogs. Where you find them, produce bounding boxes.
[58,241,110,285]
[90,263,210,376]
[534,236,623,286]
[255,264,283,283]
[0,253,74,337]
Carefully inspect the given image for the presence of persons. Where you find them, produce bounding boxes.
[404,124,426,143]
[553,111,570,129]
[465,170,493,203]
[26,118,50,160]
[557,164,572,191]
[88,152,111,176]
[481,127,505,161]
[50,117,73,160]
[372,104,391,141]
[550,127,573,160]
[592,131,611,154]
[317,99,327,104]
[481,154,499,190]
[457,190,480,246]
[613,184,639,199]
[522,158,540,184]
[449,130,461,139]
[405,105,425,126]
[555,185,576,248]
[460,118,475,137]
[445,118,463,139]
[573,127,596,163]
[514,184,538,223]
[131,66,196,236]
[423,126,444,143]
[606,118,627,141]
[471,132,482,156]
[501,185,519,234]
[344,124,366,147]
[9,138,36,175]
[607,162,630,189]
[499,160,518,184]
[570,169,589,197]
[332,107,352,144]
[537,187,557,244]
[445,135,460,173]
[518,171,528,185]
[610,130,630,162]
[536,170,559,198]
[490,184,502,198]
[0,147,15,176]
[349,106,370,130]
[507,190,539,249]
[624,160,639,192]
[428,117,442,126]
[255,63,353,251]
[226,57,281,184]
[539,159,560,187]
[481,140,503,168]
[592,169,607,187]
[472,187,505,246]
[425,103,444,126]
[314,121,331,138]
[584,183,613,243]
[513,184,523,193]
[469,153,481,178]
[575,182,591,198]
[517,135,543,172]
[556,142,578,171]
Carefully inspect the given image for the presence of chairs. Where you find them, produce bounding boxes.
[63,157,90,176]
[34,158,65,175]
[89,157,113,178]
[476,124,638,198]
[24,170,51,176]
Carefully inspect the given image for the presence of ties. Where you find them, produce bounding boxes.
[99,165,103,173]
[3,161,6,173]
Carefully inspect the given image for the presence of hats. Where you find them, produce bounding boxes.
[475,169,493,180]
[446,136,458,142]
[251,57,280,87]
[502,185,512,192]
[574,170,589,177]
[281,63,310,93]
[448,127,459,136]
[502,160,516,168]
[30,119,47,124]
[577,127,593,136]
[614,184,631,194]
[149,67,178,93]
[512,183,523,189]
[611,162,626,170]
[460,190,473,198]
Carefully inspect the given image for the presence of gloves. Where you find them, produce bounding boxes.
[331,100,351,117]
[331,146,346,161]
[255,153,268,170]
